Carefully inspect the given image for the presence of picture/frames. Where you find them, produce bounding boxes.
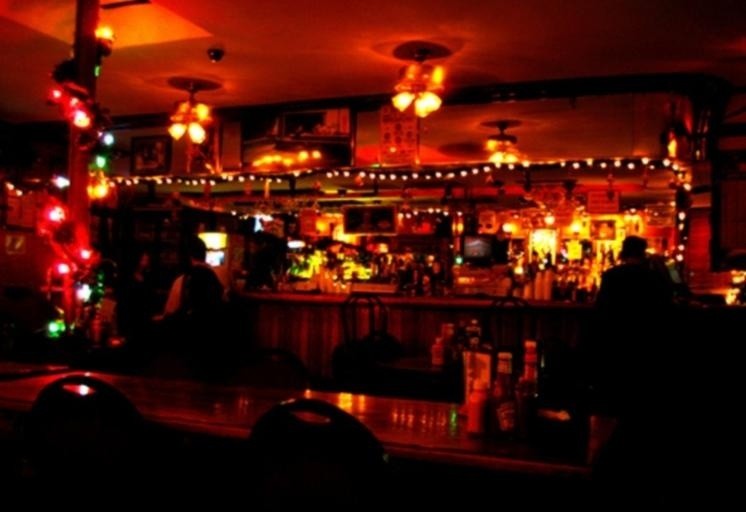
[130,135,171,175]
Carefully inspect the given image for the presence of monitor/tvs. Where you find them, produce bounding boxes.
[460,233,497,267]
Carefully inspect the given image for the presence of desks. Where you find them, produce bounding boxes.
[1,369,620,481]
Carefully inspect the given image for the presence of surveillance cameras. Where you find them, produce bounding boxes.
[208,47,223,62]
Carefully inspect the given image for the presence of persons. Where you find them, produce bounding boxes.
[586,236,671,350]
[112,232,286,383]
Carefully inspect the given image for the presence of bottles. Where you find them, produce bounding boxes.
[467,338,539,436]
[524,250,593,300]
[430,319,480,366]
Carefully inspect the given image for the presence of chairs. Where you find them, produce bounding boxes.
[17,375,168,510]
[340,294,545,393]
[241,397,391,511]
